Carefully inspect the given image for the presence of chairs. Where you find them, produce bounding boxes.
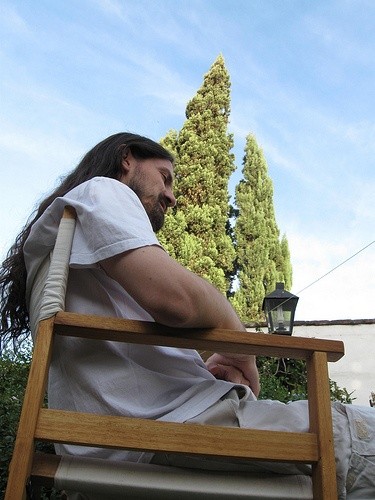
[5,205,345,500]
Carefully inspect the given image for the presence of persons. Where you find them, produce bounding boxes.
[1,132,374,500]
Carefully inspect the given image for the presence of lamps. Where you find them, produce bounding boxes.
[262,282,299,376]
